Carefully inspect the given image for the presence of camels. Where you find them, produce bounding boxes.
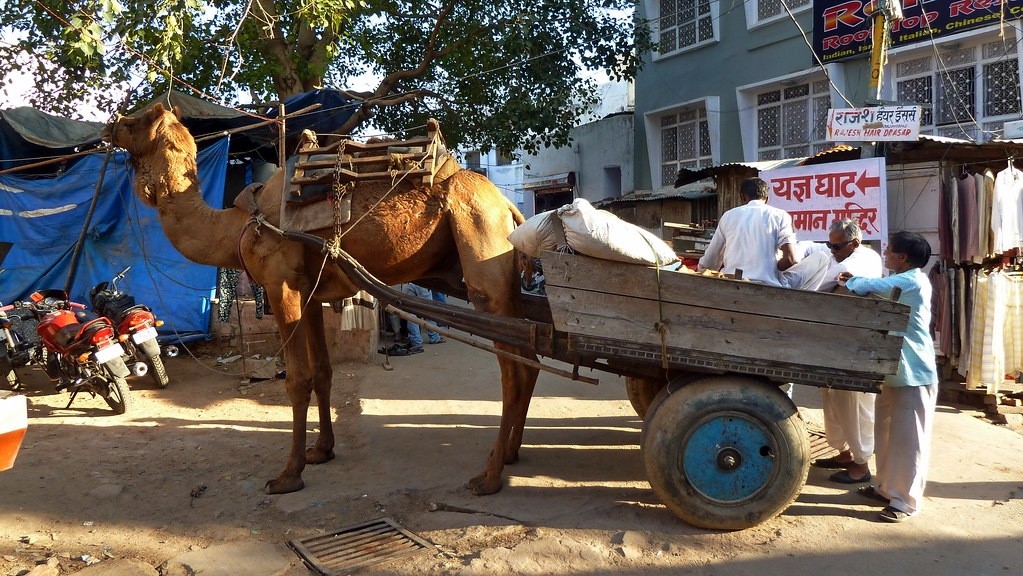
[102,103,544,497]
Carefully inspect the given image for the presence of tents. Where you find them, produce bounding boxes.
[4,90,372,348]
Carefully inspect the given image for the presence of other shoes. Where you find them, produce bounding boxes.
[815,458,852,468]
[377,331,446,356]
[830,470,871,484]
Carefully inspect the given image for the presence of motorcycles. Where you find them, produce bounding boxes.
[89,262,180,389]
[15,288,149,414]
[0,268,35,392]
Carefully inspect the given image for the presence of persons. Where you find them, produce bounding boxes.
[784,218,883,485]
[835,231,940,523]
[378,283,449,355]
[696,176,828,292]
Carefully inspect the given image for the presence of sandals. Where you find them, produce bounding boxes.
[880,506,912,523]
[858,485,890,503]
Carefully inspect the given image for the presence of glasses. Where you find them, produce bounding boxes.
[826,238,859,250]
